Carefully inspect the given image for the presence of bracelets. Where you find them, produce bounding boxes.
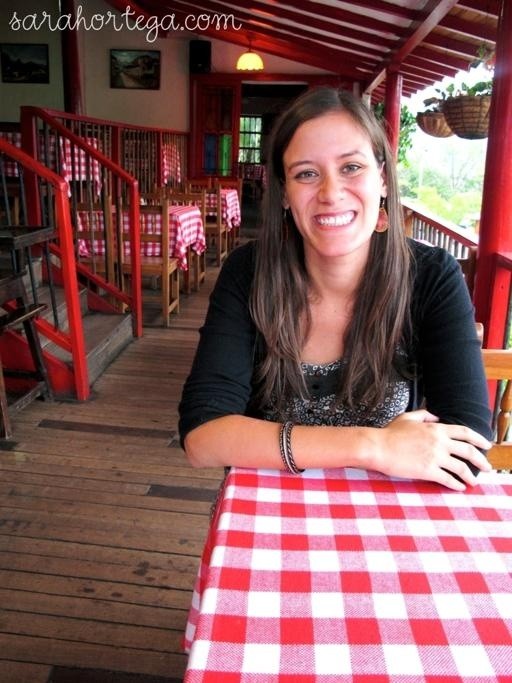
[278,419,305,476]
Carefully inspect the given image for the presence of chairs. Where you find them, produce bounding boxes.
[165,174,244,292]
[117,197,181,327]
[456,246,478,301]
[465,349,512,473]
[239,161,259,202]
[70,198,116,307]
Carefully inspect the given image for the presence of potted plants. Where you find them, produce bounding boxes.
[439,40,496,139]
[416,84,454,138]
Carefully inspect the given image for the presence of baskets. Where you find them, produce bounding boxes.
[417,112,454,137]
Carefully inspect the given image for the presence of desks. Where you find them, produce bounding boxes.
[75,203,206,294]
[122,141,182,188]
[0,225,57,442]
[1,133,102,224]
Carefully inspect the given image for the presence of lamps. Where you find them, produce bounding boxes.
[235,32,264,71]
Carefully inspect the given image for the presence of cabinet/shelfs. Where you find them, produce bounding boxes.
[179,467,512,682]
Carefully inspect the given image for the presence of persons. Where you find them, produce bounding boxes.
[177,90,496,492]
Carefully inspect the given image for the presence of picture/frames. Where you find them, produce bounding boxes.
[109,48,160,90]
[0,43,49,84]
